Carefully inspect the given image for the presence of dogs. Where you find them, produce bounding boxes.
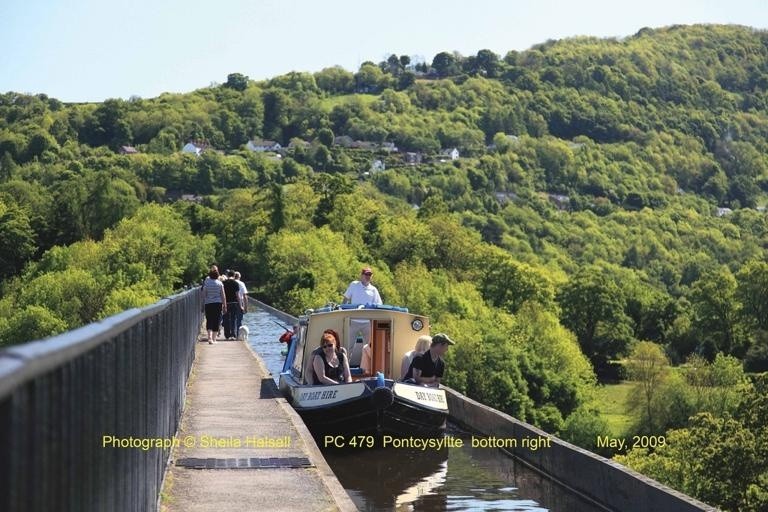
[239,324,249,340]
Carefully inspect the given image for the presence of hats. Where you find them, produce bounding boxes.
[432,334,455,345]
[362,268,372,274]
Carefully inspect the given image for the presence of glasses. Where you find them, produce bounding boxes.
[323,344,333,348]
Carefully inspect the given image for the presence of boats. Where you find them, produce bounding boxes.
[327,432,449,512]
[278,304,450,437]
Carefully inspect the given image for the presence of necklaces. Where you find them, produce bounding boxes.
[327,356,338,364]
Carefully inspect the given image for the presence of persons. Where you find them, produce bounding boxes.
[202,265,249,345]
[304,328,347,386]
[404,334,455,389]
[342,267,384,366]
[314,333,354,386]
[359,343,372,374]
[399,333,432,382]
